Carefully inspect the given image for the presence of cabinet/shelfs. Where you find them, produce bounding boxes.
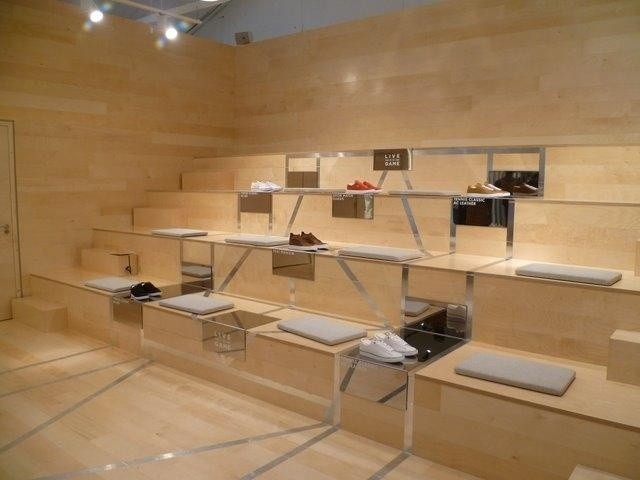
[10,144,640,474]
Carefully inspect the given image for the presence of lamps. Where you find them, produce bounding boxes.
[83,0,203,42]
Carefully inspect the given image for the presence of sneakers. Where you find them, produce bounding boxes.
[513,183,539,196]
[466,183,510,198]
[130,282,162,300]
[346,179,383,194]
[289,231,327,250]
[360,332,417,363]
[251,182,282,192]
